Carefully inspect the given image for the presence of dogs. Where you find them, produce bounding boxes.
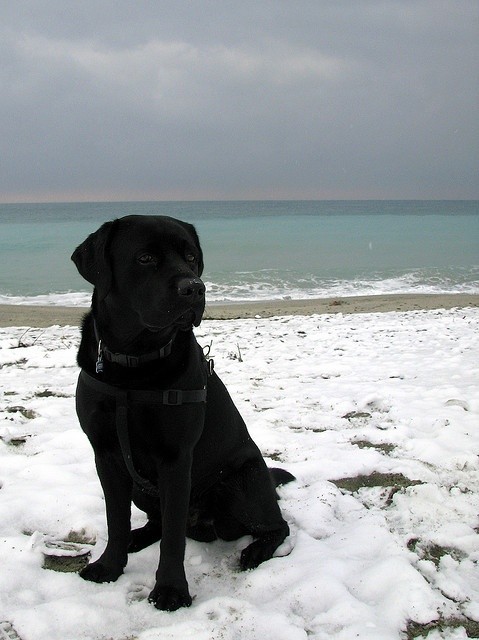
[71,215,297,612]
